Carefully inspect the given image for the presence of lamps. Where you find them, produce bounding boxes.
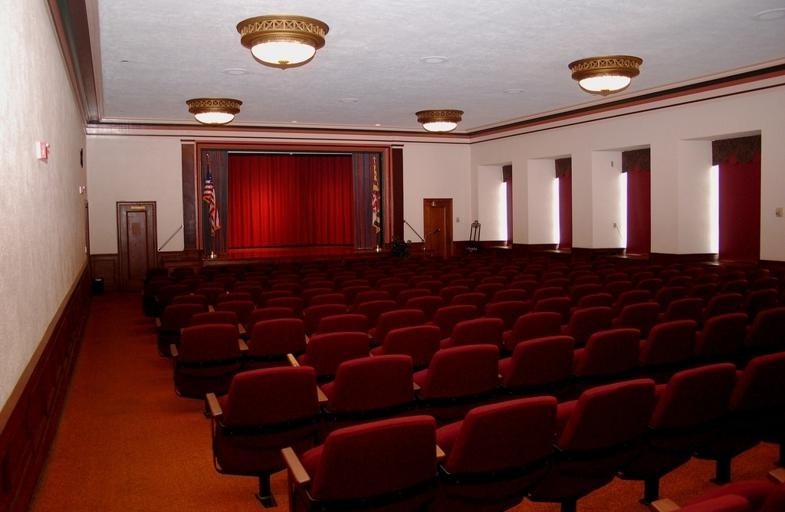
[416,110,463,133]
[184,97,243,125]
[237,15,329,71]
[568,56,643,96]
